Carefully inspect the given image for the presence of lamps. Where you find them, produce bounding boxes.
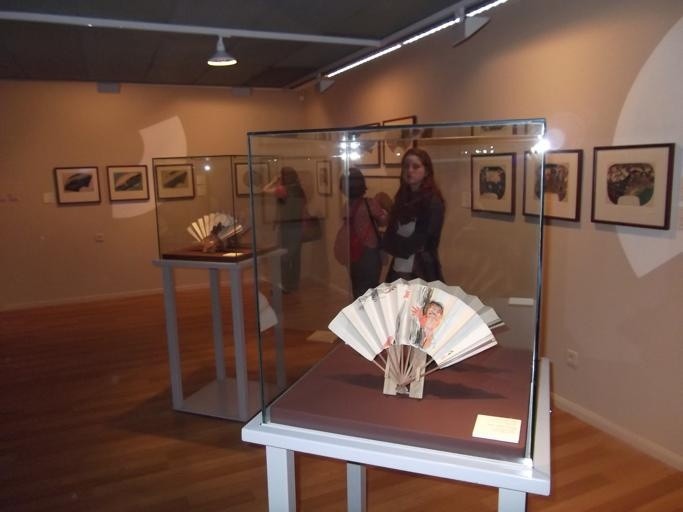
[451,7,490,47]
[317,72,336,93]
[207,35,237,67]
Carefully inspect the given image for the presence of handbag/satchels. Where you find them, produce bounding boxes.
[286,218,322,243]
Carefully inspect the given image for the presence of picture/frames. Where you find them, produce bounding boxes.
[155,163,196,201]
[316,160,332,196]
[106,165,150,201]
[234,163,271,196]
[591,143,674,230]
[383,115,416,166]
[353,123,381,167]
[470,153,516,216]
[340,175,402,220]
[522,149,583,222]
[54,167,100,205]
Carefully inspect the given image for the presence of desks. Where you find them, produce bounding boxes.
[239,340,552,511]
[152,247,288,424]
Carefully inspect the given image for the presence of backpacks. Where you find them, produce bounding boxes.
[331,221,353,266]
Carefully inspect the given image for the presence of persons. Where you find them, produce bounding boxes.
[378,148,445,285]
[340,168,392,301]
[262,166,307,295]
